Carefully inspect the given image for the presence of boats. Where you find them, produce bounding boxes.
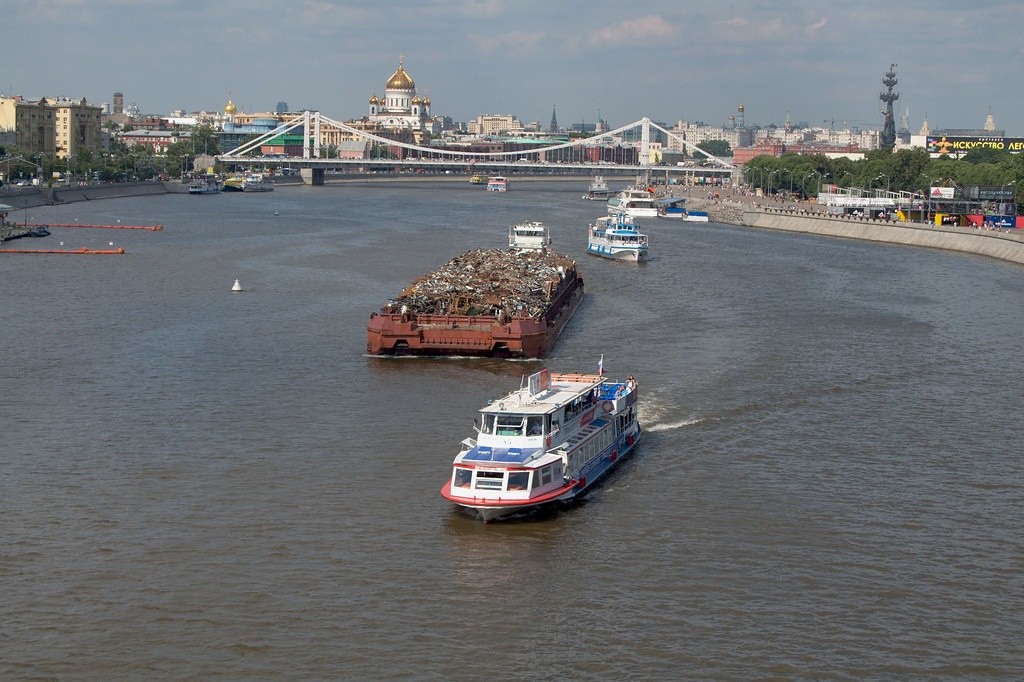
[582,173,612,201]
[470,172,489,184]
[362,246,588,364]
[440,353,643,520]
[607,185,657,217]
[486,174,511,192]
[224,169,275,192]
[190,172,223,194]
[0,203,33,241]
[507,218,552,254]
[583,202,649,263]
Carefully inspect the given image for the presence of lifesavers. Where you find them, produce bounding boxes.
[629,434,634,445]
[547,436,552,446]
[613,450,618,461]
[581,476,586,488]
